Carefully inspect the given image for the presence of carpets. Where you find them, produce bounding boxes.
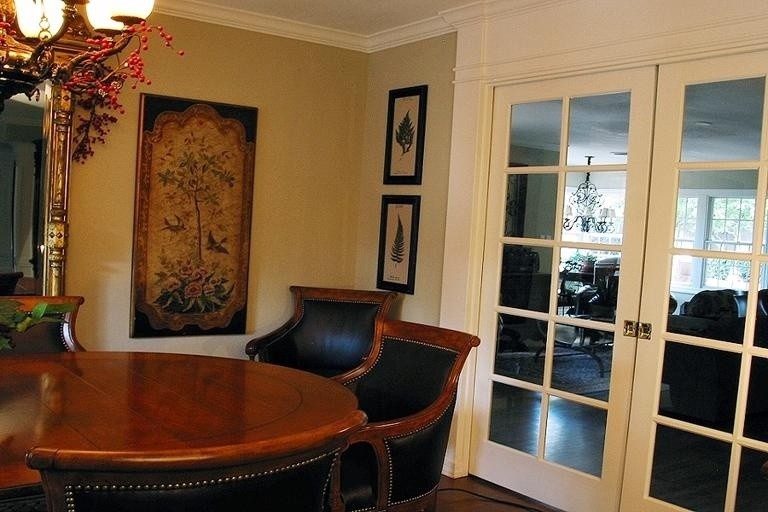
[505,351,670,393]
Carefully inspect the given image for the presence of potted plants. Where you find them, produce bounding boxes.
[580,255,597,272]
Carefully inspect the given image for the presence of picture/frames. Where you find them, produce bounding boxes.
[505,162,528,248]
[375,84,427,295]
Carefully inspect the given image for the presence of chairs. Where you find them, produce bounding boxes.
[331,318,480,510]
[0,296,88,352]
[28,411,367,511]
[245,287,394,383]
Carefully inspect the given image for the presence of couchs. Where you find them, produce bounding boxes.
[656,288,768,421]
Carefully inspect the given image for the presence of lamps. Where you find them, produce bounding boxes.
[0,0,156,97]
[562,155,616,233]
